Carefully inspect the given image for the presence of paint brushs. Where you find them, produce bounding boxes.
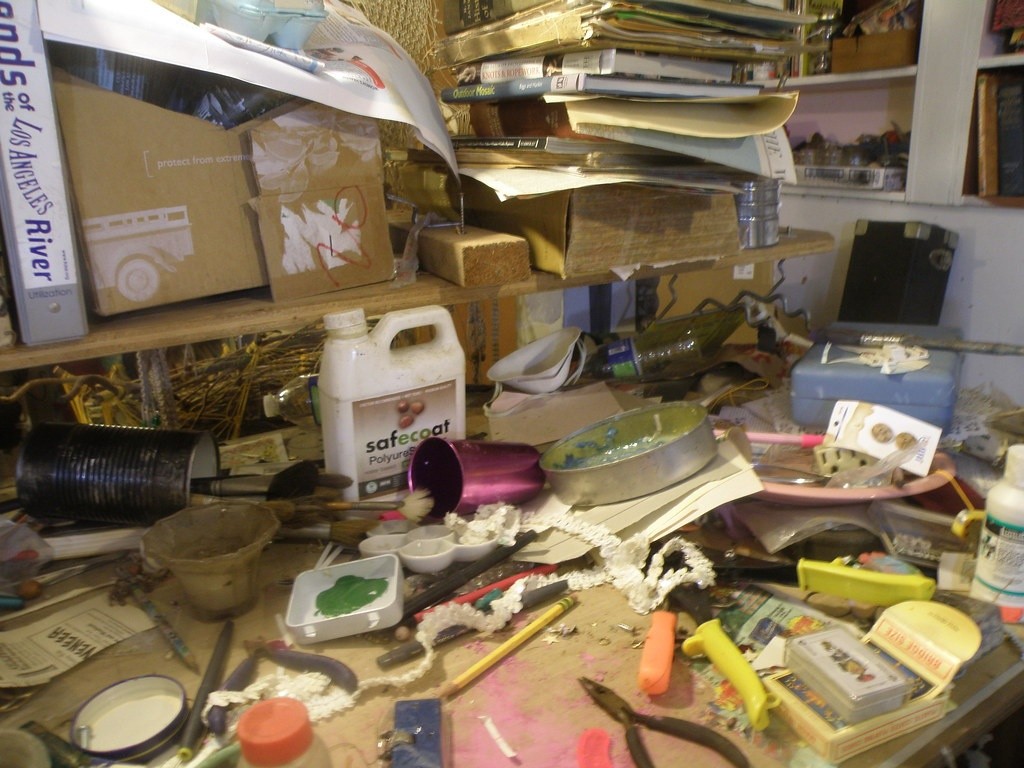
[188,456,438,551]
[822,326,1024,356]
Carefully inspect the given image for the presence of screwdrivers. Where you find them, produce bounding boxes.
[638,556,677,696]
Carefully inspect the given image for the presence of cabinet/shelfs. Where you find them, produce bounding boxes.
[936,0,1024,206]
[744,0,942,205]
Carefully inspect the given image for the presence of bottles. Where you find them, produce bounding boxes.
[311,304,471,509]
[259,371,322,432]
[237,696,332,768]
[578,329,704,382]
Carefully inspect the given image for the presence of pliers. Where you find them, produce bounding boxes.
[576,675,751,768]
[645,540,938,733]
[208,639,358,735]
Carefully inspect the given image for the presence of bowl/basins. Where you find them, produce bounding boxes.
[538,401,720,507]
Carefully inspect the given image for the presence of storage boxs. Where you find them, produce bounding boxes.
[51,65,398,324]
[791,320,966,437]
[831,27,921,75]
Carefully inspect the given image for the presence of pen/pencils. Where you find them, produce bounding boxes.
[375,528,579,701]
[712,428,829,450]
[175,617,235,763]
[124,576,202,677]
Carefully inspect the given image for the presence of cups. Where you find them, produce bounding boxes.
[405,436,545,517]
[17,420,216,522]
[140,500,282,622]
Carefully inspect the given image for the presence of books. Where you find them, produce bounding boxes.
[442,0,829,280]
[974,73,999,198]
[995,86,1023,198]
